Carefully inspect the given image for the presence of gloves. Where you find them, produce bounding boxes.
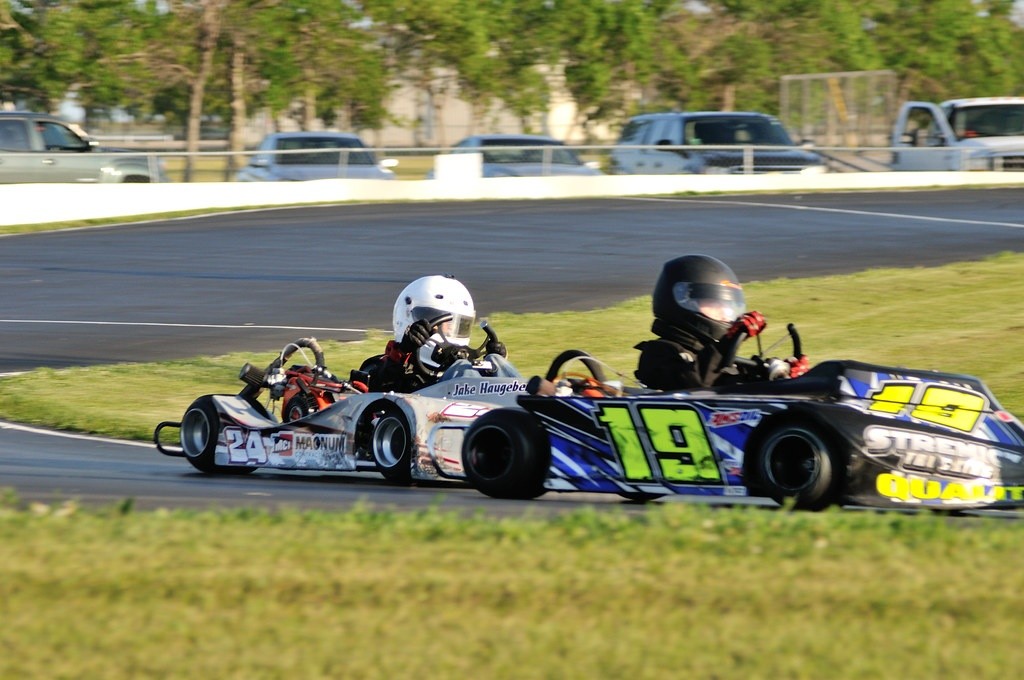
[788,356,809,377]
[484,341,507,359]
[400,318,438,354]
[727,311,766,337]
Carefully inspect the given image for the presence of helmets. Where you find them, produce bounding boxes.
[393,272,476,377]
[652,255,747,340]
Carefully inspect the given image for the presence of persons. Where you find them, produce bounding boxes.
[349,275,522,394]
[633,255,809,393]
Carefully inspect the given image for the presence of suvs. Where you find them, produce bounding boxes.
[613,111,821,175]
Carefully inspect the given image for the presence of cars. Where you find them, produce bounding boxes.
[423,135,600,179]
[889,97,1024,172]
[231,131,393,181]
[0,111,166,184]
